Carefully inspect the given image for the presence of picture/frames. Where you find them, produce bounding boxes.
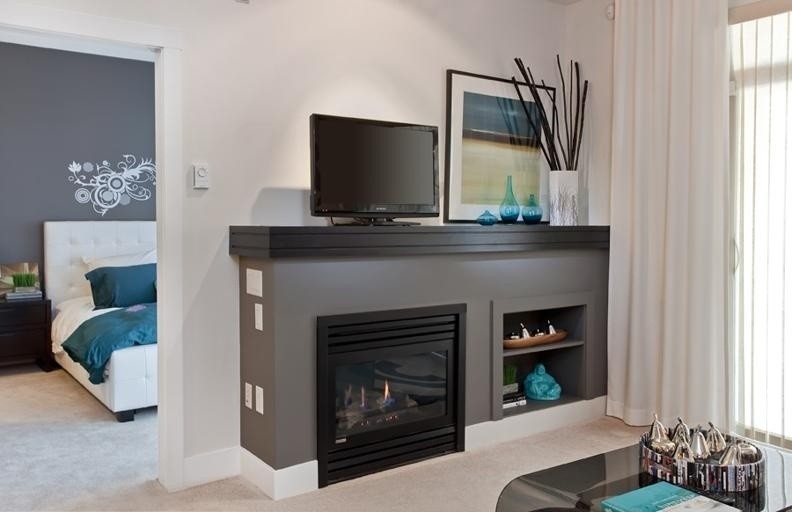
[443,68,557,224]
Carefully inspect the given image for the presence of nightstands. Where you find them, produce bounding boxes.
[0,297,55,373]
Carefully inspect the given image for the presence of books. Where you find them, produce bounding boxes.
[600,480,744,512]
[503,393,526,408]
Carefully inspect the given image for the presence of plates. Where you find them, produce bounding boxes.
[639,428,765,493]
[503,329,568,349]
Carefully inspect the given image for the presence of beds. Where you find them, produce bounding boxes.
[43,220,157,422]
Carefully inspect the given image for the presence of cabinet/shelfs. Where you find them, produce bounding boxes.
[228,223,611,500]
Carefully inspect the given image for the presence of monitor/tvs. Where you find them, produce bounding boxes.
[310,114,440,225]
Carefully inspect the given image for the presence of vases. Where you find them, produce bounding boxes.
[548,170,580,226]
[521,194,543,224]
[477,209,498,225]
[499,174,521,223]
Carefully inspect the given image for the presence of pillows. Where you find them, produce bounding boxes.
[84,262,156,311]
